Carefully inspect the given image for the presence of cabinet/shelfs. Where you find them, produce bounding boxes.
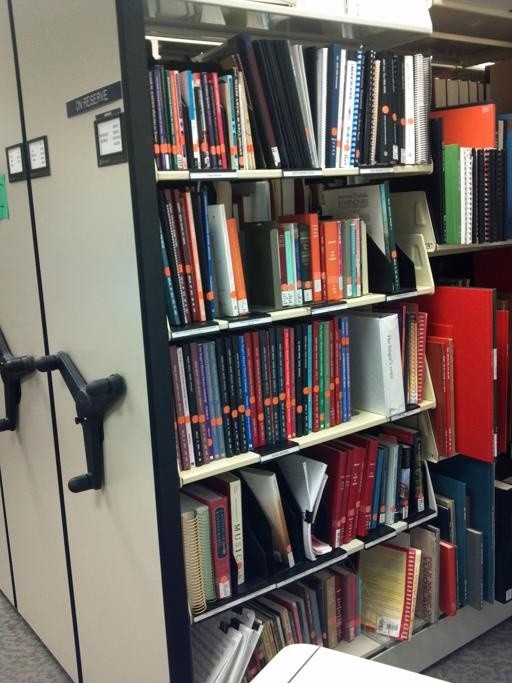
[1,0,512,683]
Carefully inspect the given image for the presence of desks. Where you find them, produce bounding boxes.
[249,643,448,683]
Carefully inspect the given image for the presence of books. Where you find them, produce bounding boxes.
[169,300,422,683]
[147,33,432,171]
[379,279,511,612]
[156,181,400,327]
[370,77,512,245]
